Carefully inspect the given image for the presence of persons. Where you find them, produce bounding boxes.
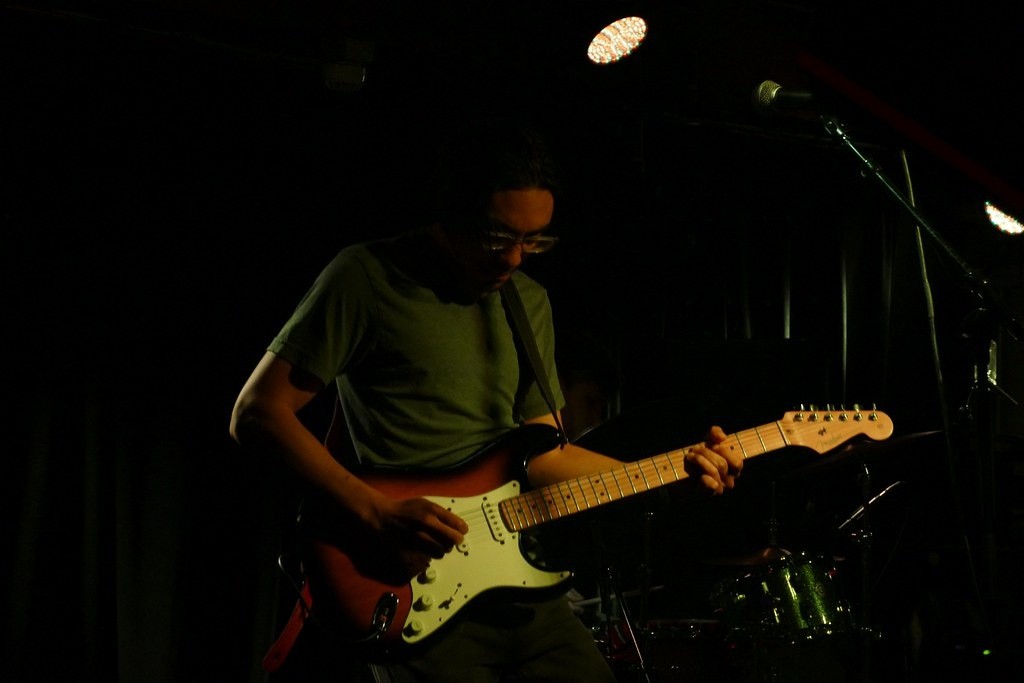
[229,116,742,683]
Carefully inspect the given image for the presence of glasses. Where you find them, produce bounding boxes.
[474,225,557,255]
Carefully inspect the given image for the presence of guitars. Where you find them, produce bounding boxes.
[292,401,900,667]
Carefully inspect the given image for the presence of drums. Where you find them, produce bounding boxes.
[718,551,845,646]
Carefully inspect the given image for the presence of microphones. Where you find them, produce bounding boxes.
[749,80,839,120]
